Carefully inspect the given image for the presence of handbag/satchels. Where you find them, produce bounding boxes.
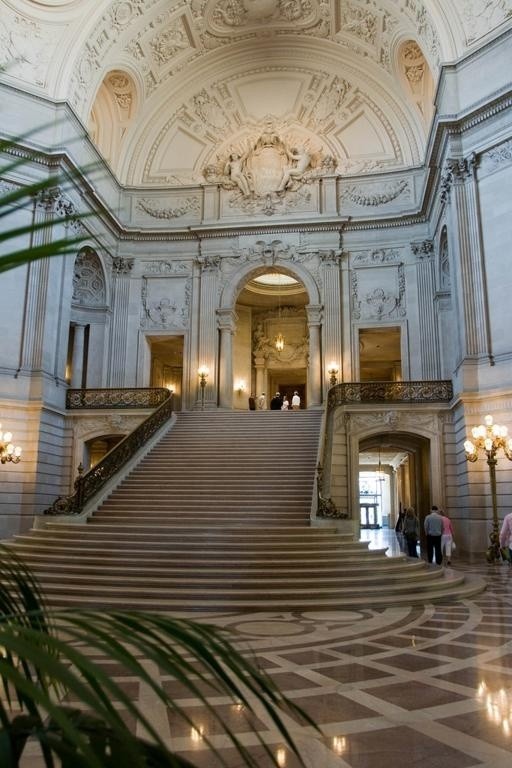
[452,542,456,552]
[404,532,416,541]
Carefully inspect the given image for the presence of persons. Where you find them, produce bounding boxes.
[402,509,419,559]
[498,512,512,559]
[423,506,442,565]
[248,391,301,410]
[440,510,455,567]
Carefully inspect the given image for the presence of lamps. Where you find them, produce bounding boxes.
[326,359,339,384]
[235,379,246,395]
[0,420,25,469]
[274,274,286,353]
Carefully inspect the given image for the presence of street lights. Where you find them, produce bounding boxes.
[0,423,22,465]
[327,359,341,390]
